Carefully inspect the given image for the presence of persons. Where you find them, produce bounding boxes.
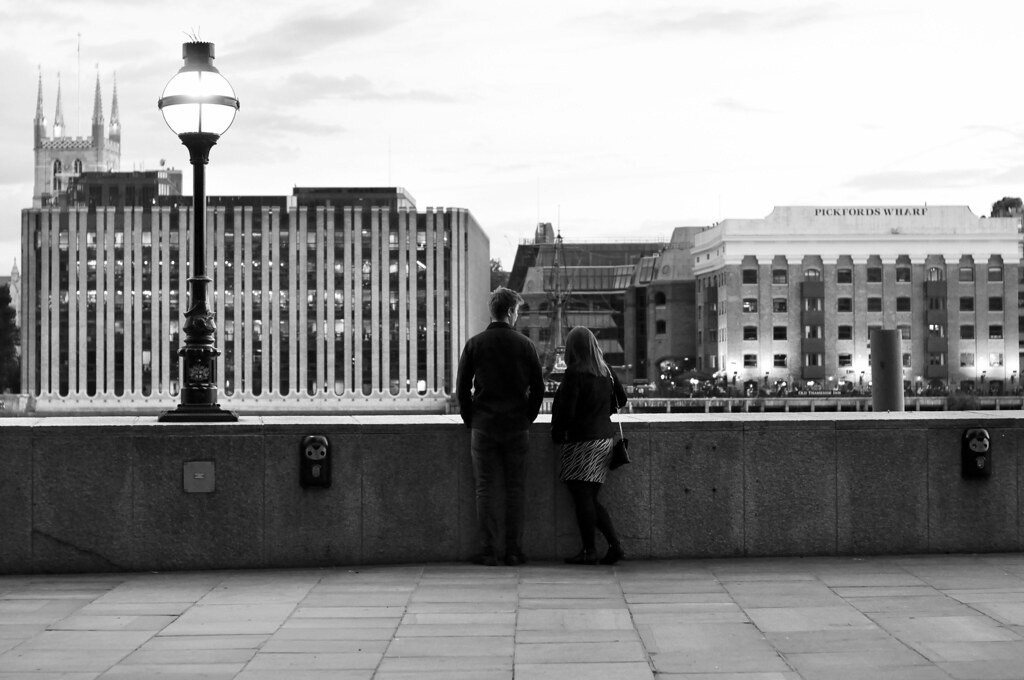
[456,286,545,567]
[548,326,628,564]
[657,371,1024,397]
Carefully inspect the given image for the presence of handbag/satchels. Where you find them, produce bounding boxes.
[609,438,632,471]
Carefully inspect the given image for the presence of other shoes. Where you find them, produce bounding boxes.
[471,553,498,566]
[599,540,624,565]
[505,547,526,566]
[565,545,597,565]
